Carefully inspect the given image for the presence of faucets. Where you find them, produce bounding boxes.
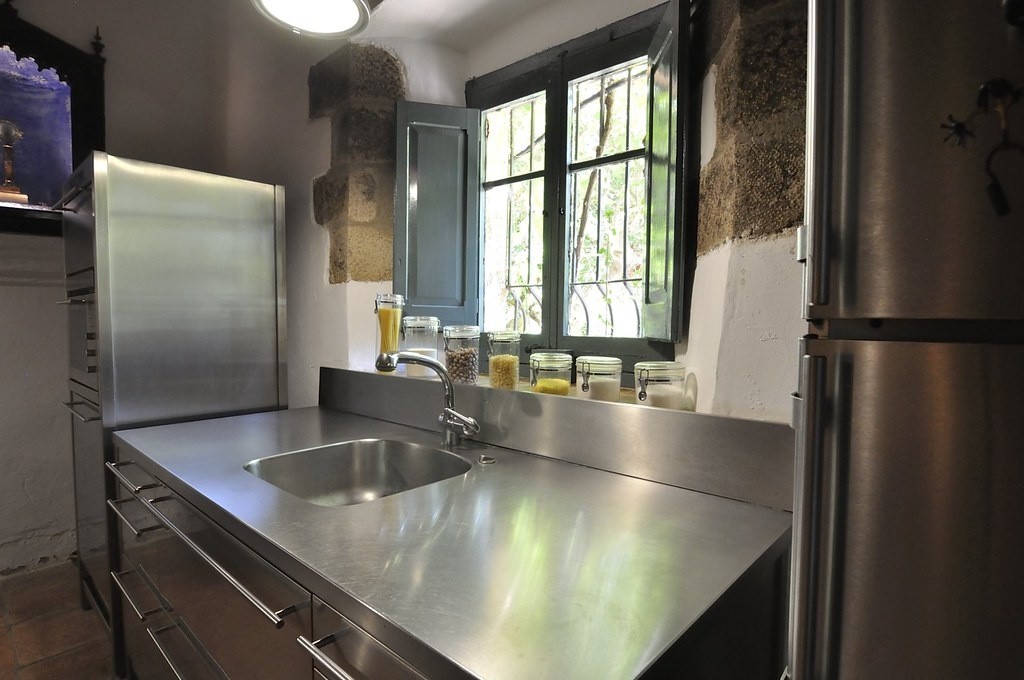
[372,351,455,411]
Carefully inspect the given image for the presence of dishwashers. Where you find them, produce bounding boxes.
[111,404,793,680]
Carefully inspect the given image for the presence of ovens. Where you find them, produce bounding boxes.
[52,149,293,429]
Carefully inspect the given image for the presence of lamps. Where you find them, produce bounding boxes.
[253,1,385,43]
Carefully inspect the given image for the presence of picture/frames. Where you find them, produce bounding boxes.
[0,0,112,236]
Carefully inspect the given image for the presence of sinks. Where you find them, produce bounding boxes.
[241,427,476,508]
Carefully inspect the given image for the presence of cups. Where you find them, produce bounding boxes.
[486,329,520,391]
[576,356,623,401]
[634,361,687,412]
[375,293,404,373]
[403,315,439,378]
[529,352,572,396]
[443,324,481,385]
